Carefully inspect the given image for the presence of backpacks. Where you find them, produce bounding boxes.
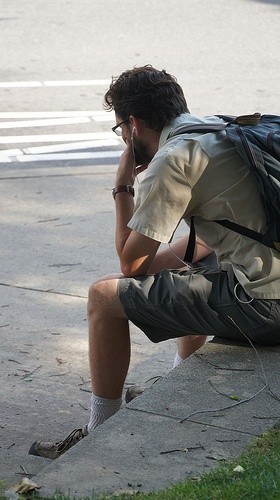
[166,112,280,264]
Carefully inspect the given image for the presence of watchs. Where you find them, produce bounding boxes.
[112,180,135,200]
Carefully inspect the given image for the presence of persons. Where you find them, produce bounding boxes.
[27,65,280,459]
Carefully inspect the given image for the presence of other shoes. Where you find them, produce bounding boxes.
[125,376,165,404]
[26,425,89,458]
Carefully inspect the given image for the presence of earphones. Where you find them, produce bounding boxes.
[132,127,136,132]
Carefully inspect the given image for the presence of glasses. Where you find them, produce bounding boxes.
[111,117,133,137]
